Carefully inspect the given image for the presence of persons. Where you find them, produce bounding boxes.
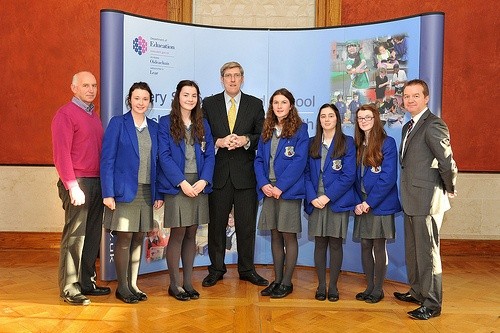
[393,78,458,320]
[350,105,402,304]
[343,39,371,94]
[334,93,362,124]
[51,70,110,305]
[374,63,407,99]
[255,88,310,298]
[200,62,269,287]
[303,103,361,302]
[372,33,408,67]
[372,95,405,126]
[157,80,214,300]
[101,81,165,304]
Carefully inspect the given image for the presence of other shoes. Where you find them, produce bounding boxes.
[168,285,190,301]
[364,289,385,303]
[328,288,339,302]
[182,285,200,300]
[129,286,148,300]
[270,283,293,298]
[315,286,326,301]
[356,292,368,301]
[261,281,281,296]
[115,287,139,304]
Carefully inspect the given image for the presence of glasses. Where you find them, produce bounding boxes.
[357,116,375,122]
[223,73,242,78]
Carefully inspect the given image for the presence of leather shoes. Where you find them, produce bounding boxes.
[202,273,223,287]
[81,286,111,296]
[239,271,269,286]
[407,306,441,320]
[59,293,91,305]
[394,291,423,306]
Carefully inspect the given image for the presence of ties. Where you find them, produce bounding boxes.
[227,98,237,134]
[407,119,414,136]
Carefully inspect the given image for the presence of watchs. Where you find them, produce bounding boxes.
[244,136,250,145]
